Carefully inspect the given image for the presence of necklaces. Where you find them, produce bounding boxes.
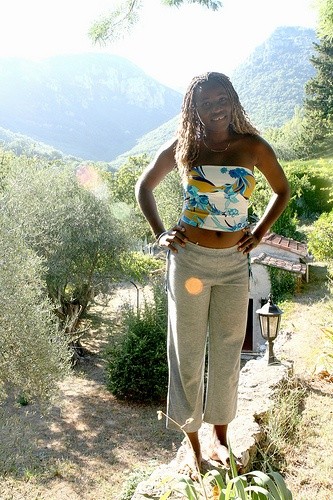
[201,133,233,152]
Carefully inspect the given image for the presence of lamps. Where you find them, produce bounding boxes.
[255,292,285,366]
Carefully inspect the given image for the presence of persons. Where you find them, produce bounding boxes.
[134,71,291,481]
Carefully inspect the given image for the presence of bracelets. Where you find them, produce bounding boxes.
[156,231,166,243]
